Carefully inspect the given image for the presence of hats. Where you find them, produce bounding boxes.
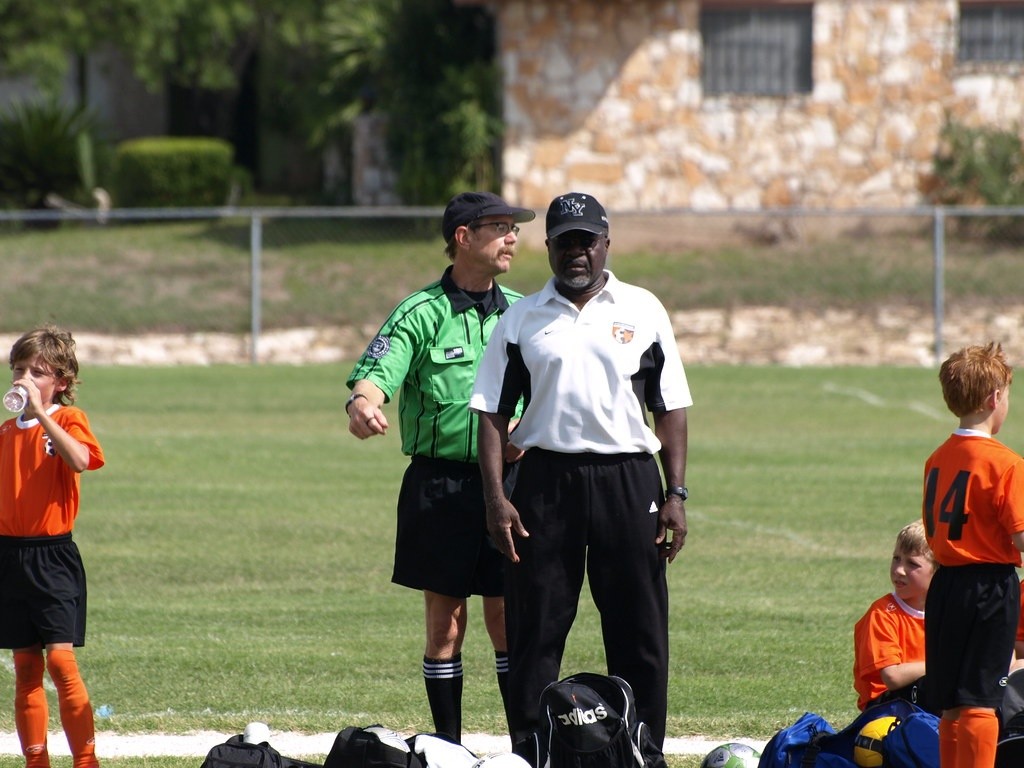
[442,191,536,243]
[546,192,608,239]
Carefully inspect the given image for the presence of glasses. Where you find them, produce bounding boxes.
[482,222,519,236]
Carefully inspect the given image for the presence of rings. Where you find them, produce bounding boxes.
[366,417,374,425]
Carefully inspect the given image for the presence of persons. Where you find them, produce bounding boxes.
[0,321,105,767]
[467,193,694,768]
[346,192,535,757]
[852,342,1024,768]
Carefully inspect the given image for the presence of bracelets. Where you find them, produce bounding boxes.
[345,393,368,417]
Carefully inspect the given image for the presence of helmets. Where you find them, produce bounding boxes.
[854,716,903,767]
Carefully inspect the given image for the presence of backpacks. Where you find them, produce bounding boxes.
[758,712,837,768]
[199,734,299,768]
[996,667,1024,768]
[813,697,942,768]
[323,723,422,768]
[516,672,668,768]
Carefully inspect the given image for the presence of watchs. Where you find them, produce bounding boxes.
[666,486,689,500]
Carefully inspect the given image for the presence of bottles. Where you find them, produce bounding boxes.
[95,704,111,717]
[3,385,28,413]
